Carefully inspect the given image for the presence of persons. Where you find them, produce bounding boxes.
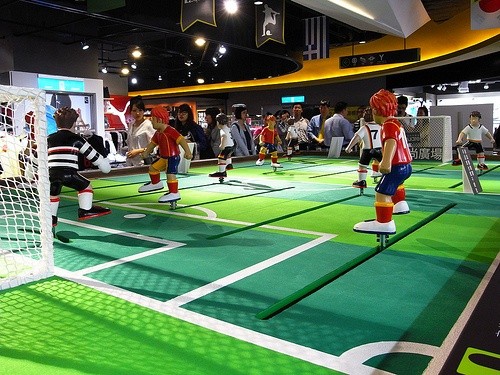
[208,113,235,177]
[452,110,494,171]
[414,106,430,146]
[119,95,154,166]
[345,108,383,188]
[205,103,290,156]
[395,96,414,141]
[353,89,412,235]
[286,99,366,150]
[128,107,193,202]
[25,106,112,226]
[255,115,283,167]
[0,124,23,186]
[175,104,208,160]
[283,119,300,157]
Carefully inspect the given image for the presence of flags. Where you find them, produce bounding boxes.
[471,0,500,30]
[302,16,330,61]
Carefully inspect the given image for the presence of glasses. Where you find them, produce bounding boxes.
[177,111,188,115]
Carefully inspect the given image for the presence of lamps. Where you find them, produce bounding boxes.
[81,38,227,85]
[437,82,489,91]
